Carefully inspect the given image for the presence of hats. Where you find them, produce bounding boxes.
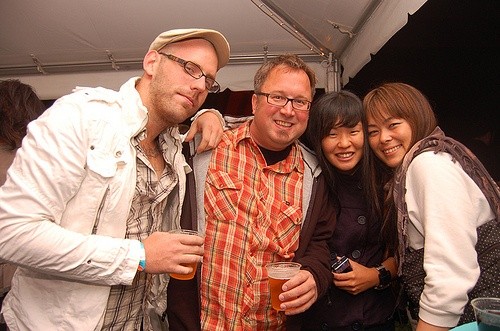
[148,29,230,73]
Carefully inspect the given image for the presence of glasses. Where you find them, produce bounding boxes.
[157,52,221,94]
[256,92,312,111]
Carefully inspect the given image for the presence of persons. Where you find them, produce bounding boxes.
[306,91,398,331]
[0,80,46,302]
[363,84,500,331]
[0,28,226,331]
[168,55,337,331]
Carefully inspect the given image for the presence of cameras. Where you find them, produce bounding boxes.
[331,256,352,274]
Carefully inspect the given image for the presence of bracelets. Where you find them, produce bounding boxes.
[138,243,145,272]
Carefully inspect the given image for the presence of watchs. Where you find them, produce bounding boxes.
[375,264,392,286]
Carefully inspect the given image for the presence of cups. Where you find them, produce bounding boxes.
[265,262,302,312]
[471,298,500,331]
[167,229,206,280]
